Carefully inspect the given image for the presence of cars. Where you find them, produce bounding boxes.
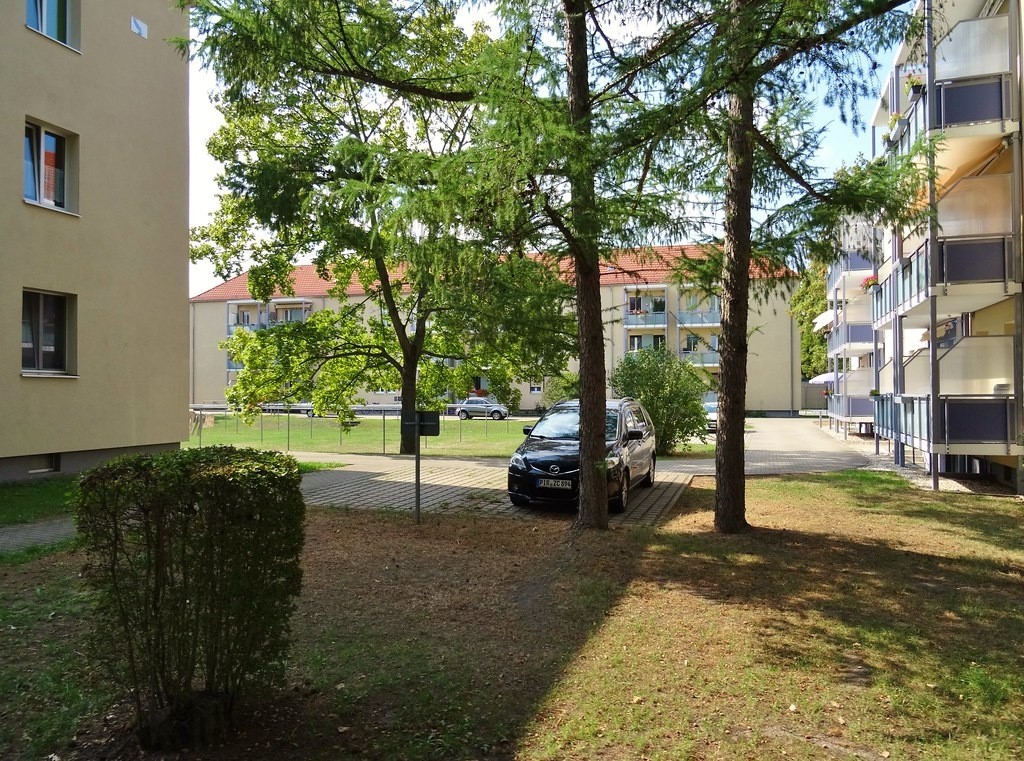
[701,402,718,432]
[455,397,509,421]
[301,409,342,418]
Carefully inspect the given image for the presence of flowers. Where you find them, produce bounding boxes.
[828,391,833,394]
[822,330,831,335]
[888,111,905,130]
[629,309,649,313]
[822,391,829,396]
[904,72,922,95]
[859,275,878,290]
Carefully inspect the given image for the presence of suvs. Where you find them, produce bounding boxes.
[507,397,657,514]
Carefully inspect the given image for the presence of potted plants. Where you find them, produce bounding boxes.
[881,133,894,152]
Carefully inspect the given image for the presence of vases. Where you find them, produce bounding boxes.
[907,85,922,102]
[832,395,838,399]
[869,283,880,295]
[868,389,883,402]
[825,395,829,399]
[894,396,912,404]
[891,257,910,271]
[824,334,829,338]
[829,394,832,397]
[890,119,909,141]
